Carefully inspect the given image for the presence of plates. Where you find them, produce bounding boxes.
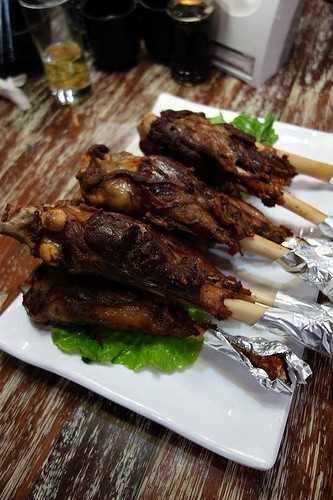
[0,91,333,472]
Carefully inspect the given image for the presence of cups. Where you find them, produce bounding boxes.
[18,0,93,105]
[79,0,137,72]
[139,0,170,65]
[166,0,216,87]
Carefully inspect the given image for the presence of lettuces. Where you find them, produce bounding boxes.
[205,112,279,201]
[50,307,210,375]
